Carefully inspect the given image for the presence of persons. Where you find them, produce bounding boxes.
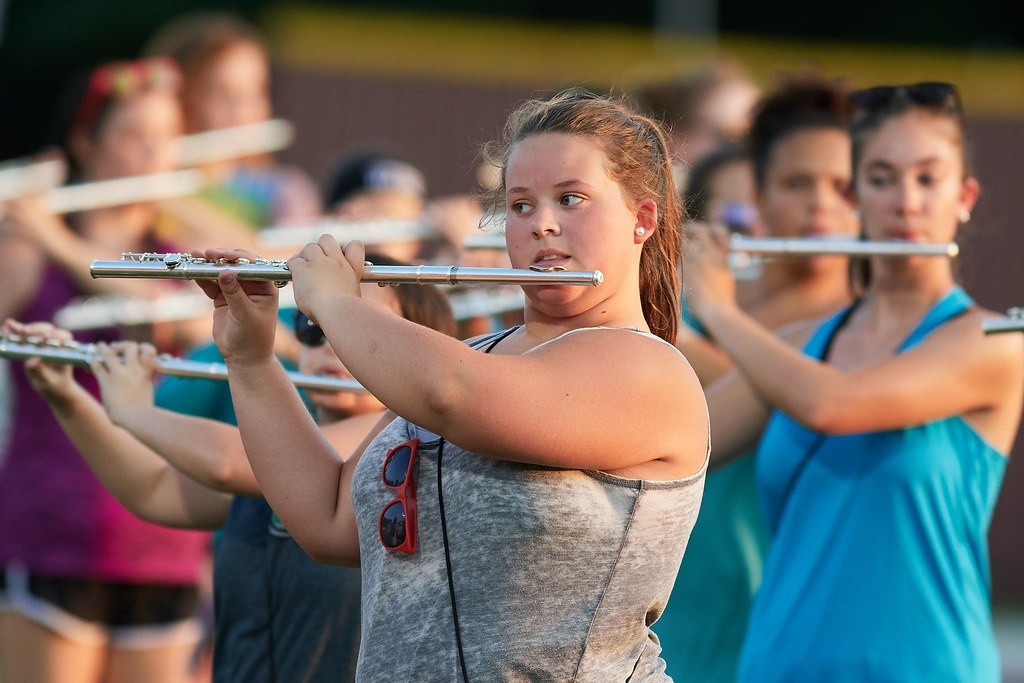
[192,87,713,683]
[640,73,1024,683]
[0,25,526,683]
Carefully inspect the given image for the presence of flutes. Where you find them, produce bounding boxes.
[730,233,963,261]
[44,169,210,216]
[87,246,605,289]
[0,119,295,196]
[983,305,1023,337]
[1,332,370,396]
[257,212,505,250]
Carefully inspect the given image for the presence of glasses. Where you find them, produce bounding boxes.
[294,308,326,347]
[712,202,760,240]
[846,80,968,147]
[378,438,421,554]
[78,56,180,141]
